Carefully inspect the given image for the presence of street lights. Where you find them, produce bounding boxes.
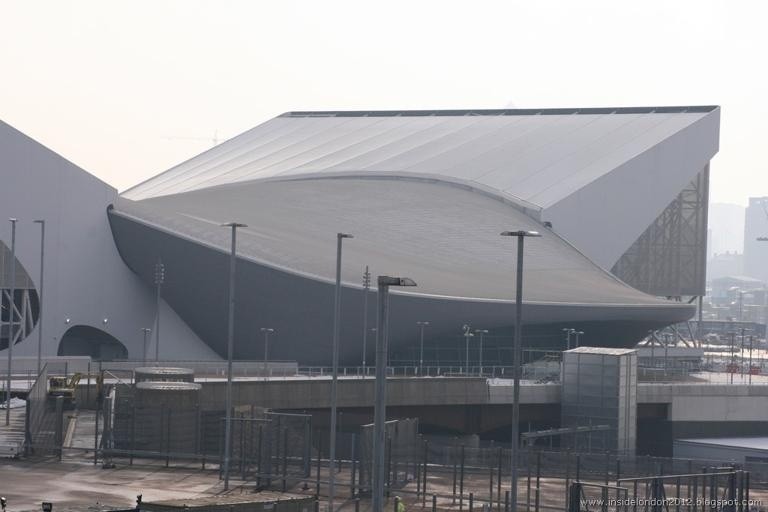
[259,328,274,375]
[153,252,164,367]
[220,221,248,491]
[648,329,673,376]
[34,219,45,376]
[562,328,584,350]
[475,330,489,378]
[363,265,371,379]
[499,229,542,512]
[327,232,354,512]
[464,333,473,377]
[6,218,20,426]
[727,327,757,386]
[417,321,429,377]
[140,328,151,366]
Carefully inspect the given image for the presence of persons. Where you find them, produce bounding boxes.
[394,496,405,512]
